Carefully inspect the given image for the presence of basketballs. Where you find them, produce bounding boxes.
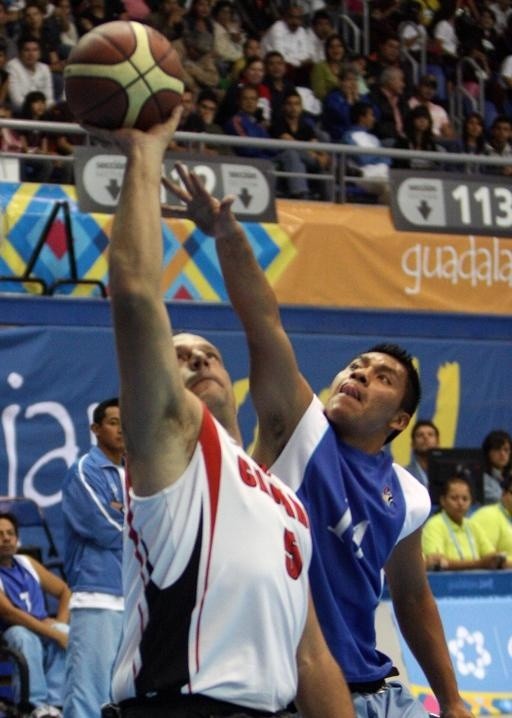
[65,21,186,130]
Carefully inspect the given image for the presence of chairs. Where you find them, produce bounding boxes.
[1,497,65,591]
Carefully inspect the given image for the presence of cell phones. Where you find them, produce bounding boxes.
[487,552,505,569]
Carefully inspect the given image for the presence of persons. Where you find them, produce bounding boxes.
[2,0,512,203]
[469,470,512,568]
[467,431,512,505]
[0,512,73,718]
[81,105,356,718]
[158,162,474,718]
[403,420,438,488]
[421,478,507,570]
[60,396,129,718]
